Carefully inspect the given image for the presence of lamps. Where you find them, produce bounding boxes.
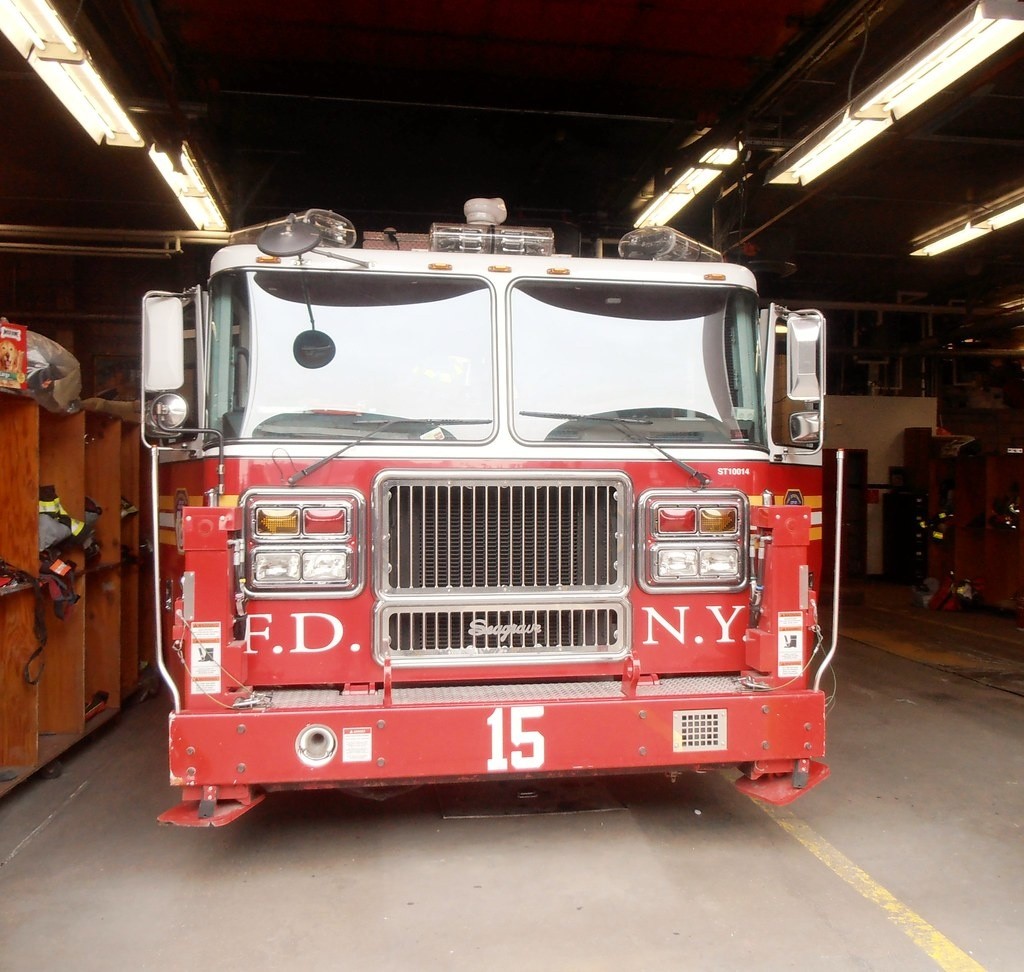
[150,142,230,231]
[0,0,145,148]
[762,0,1024,186]
[632,141,745,232]
[908,187,1024,257]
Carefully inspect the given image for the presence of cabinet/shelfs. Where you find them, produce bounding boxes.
[0,380,159,801]
[916,439,1024,614]
[936,296,1023,441]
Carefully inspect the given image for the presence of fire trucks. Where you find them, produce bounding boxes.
[138,198,845,825]
